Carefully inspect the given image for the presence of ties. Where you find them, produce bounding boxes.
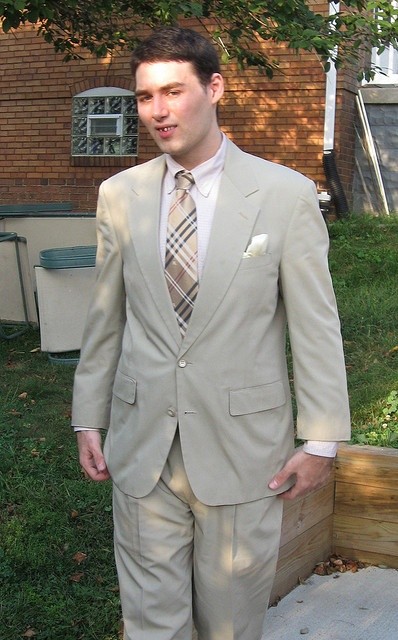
[162,168,200,342]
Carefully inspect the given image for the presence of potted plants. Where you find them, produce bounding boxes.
[48,350,79,369]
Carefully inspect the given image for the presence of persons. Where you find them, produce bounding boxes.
[69,25,353,638]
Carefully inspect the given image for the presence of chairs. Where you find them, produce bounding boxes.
[263,443,398,640]
